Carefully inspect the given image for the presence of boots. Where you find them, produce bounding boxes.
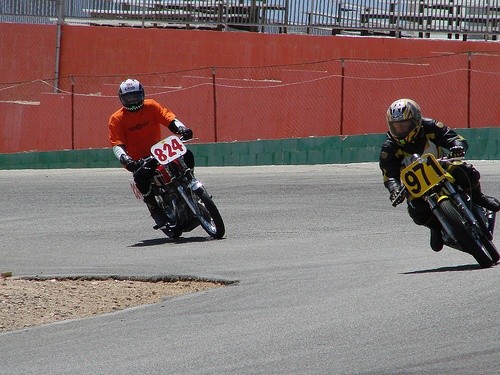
[424,213,443,252]
[471,182,500,212]
[144,195,167,226]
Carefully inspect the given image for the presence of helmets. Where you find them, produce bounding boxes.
[386,98,422,145]
[118,79,145,112]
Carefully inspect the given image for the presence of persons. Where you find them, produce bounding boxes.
[108,79,193,230]
[380,99,500,252]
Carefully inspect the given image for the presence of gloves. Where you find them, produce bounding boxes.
[389,185,406,205]
[447,145,464,159]
[126,159,144,172]
[182,128,193,140]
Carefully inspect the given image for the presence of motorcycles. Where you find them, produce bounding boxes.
[391,153,499,268]
[132,134,226,240]
